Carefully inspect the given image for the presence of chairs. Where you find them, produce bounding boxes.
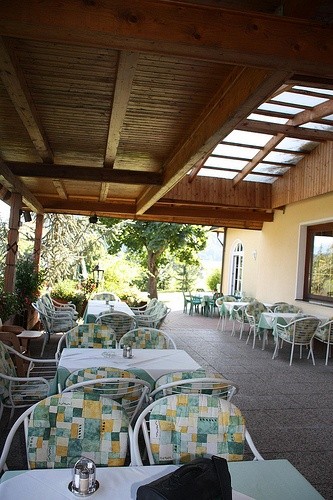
[0,293,177,475]
[182,290,333,366]
[150,378,239,402]
[133,393,264,466]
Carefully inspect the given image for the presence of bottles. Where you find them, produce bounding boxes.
[123,345,132,357]
[74,465,94,495]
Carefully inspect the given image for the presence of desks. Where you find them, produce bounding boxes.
[220,302,275,332]
[49,348,204,396]
[0,459,326,500]
[83,300,136,323]
[257,312,330,351]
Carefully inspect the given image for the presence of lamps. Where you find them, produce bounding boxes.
[19,209,31,222]
[89,210,98,224]
